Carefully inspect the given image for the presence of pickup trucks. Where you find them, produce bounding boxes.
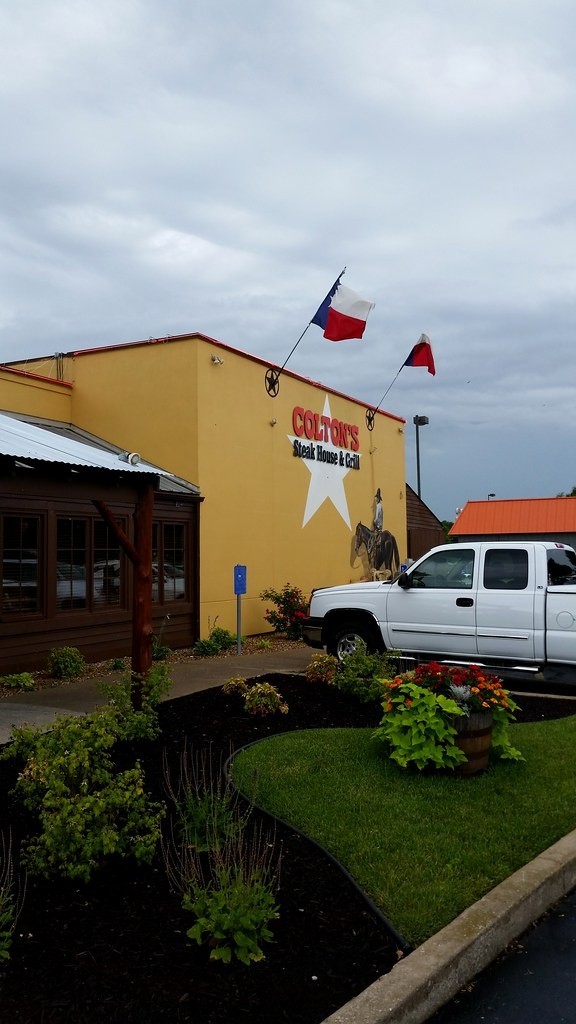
[300,541,576,703]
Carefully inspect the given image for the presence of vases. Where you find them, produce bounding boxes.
[403,708,491,778]
[288,624,301,640]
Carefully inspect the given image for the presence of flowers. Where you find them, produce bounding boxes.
[377,660,527,770]
[260,580,308,633]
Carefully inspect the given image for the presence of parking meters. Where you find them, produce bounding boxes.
[233,564,247,657]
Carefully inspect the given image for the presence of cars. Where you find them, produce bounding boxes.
[2,559,186,610]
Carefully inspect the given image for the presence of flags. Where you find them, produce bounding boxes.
[404,334,435,376]
[310,275,376,342]
